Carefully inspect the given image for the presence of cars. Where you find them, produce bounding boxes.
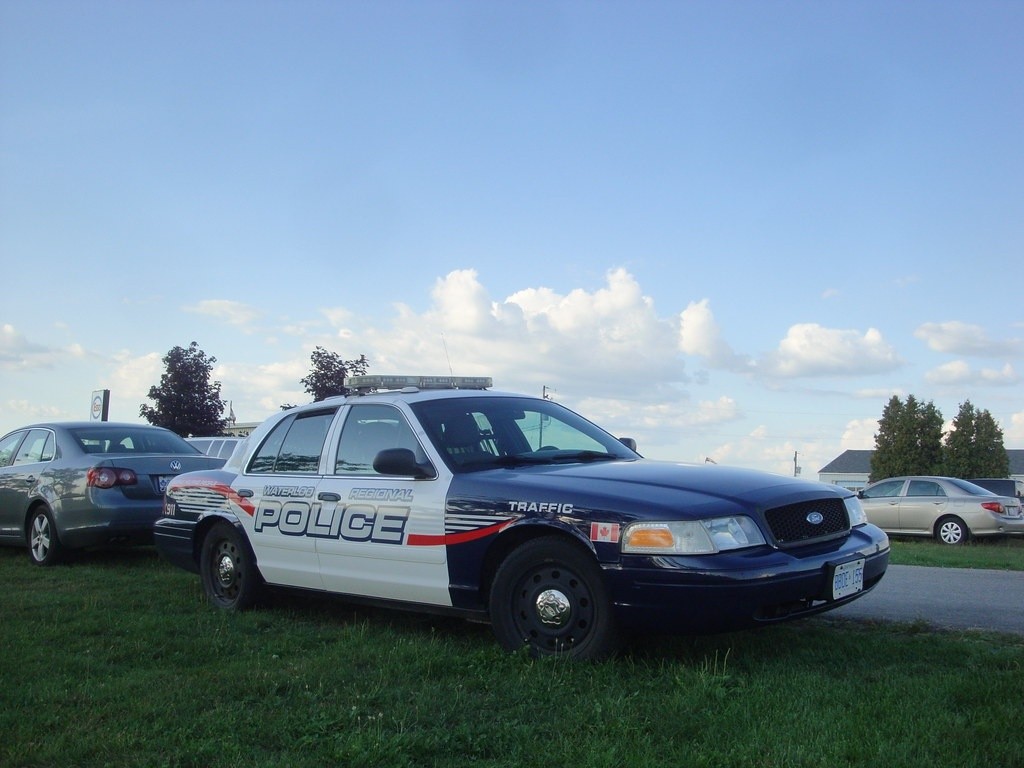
[154,373,890,658]
[852,472,1024,545]
[0,423,229,563]
